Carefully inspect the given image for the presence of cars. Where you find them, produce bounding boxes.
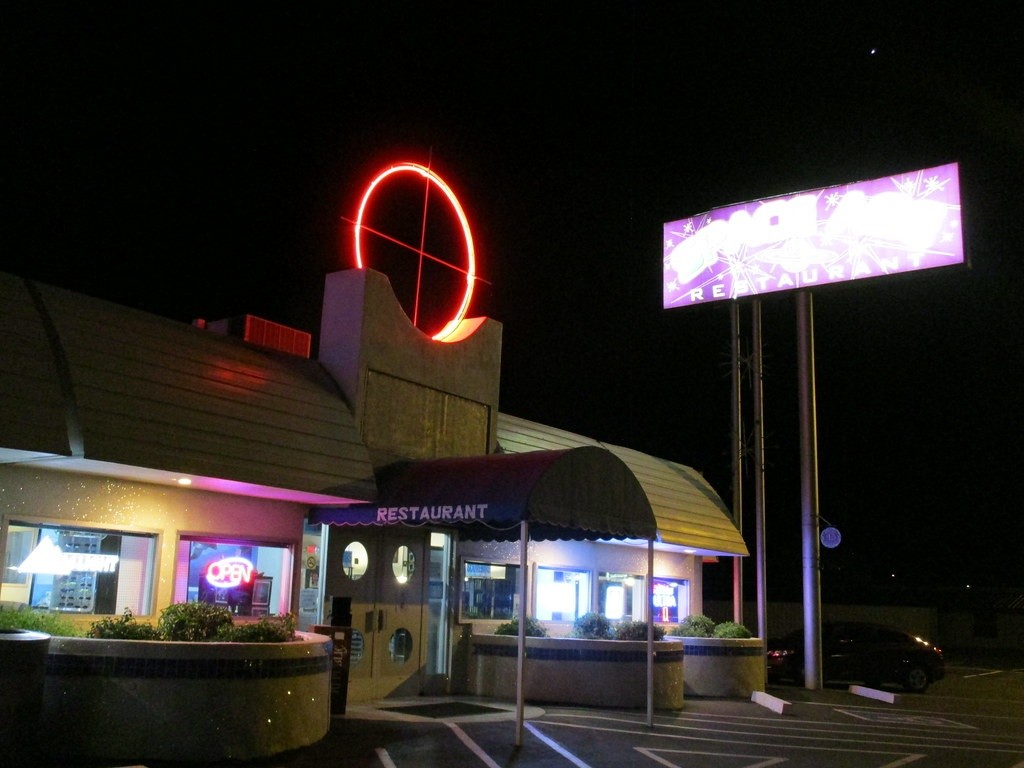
[767,621,946,694]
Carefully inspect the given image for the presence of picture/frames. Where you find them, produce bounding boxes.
[251,579,272,606]
[251,607,270,617]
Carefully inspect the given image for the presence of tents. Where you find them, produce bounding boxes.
[308,445,752,747]
